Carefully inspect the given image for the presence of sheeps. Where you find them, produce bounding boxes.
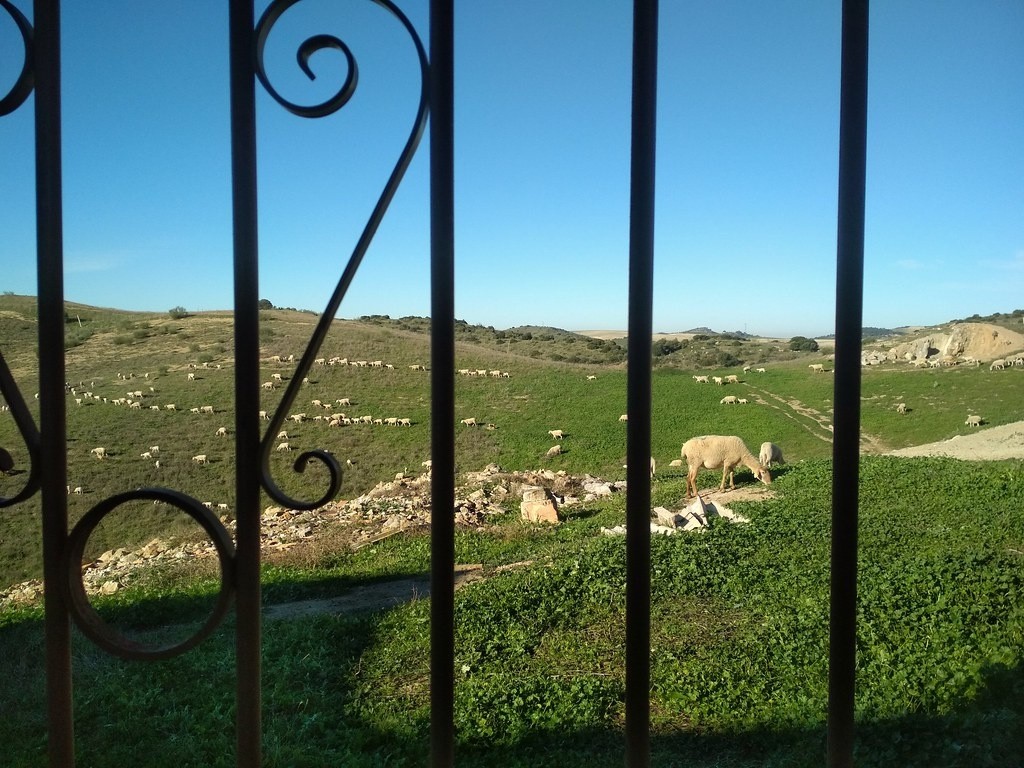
[461,418,477,427]
[0,362,271,512]
[808,364,826,373]
[323,413,373,428]
[311,399,332,410]
[623,457,655,478]
[261,382,276,391]
[884,349,1024,431]
[545,429,564,458]
[291,413,321,423]
[422,460,432,469]
[409,364,426,371]
[347,459,353,467]
[458,368,509,378]
[619,414,628,422]
[336,398,351,407]
[373,418,412,427]
[277,431,291,452]
[680,435,785,499]
[315,357,395,370]
[270,374,282,380]
[270,355,294,363]
[302,378,309,383]
[587,375,596,381]
[692,367,767,404]
